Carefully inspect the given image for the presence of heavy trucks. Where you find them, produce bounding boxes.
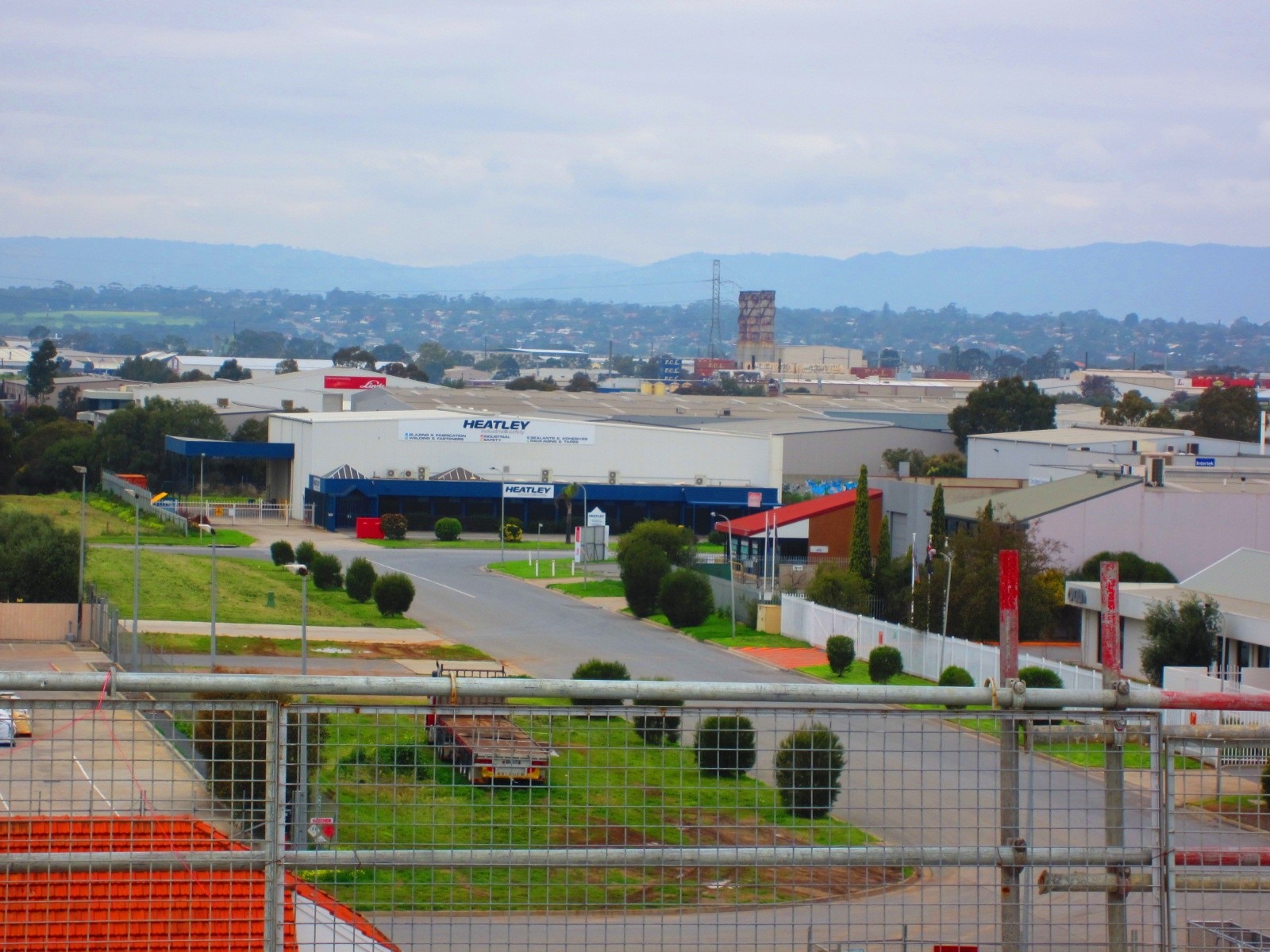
[425,661,548,785]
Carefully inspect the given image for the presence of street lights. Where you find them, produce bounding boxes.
[124,488,139,673]
[199,453,205,523]
[535,524,544,577]
[1198,601,1227,802]
[284,564,308,704]
[931,549,952,683]
[198,524,216,674]
[572,482,586,527]
[73,466,87,641]
[711,512,736,639]
[489,467,504,566]
